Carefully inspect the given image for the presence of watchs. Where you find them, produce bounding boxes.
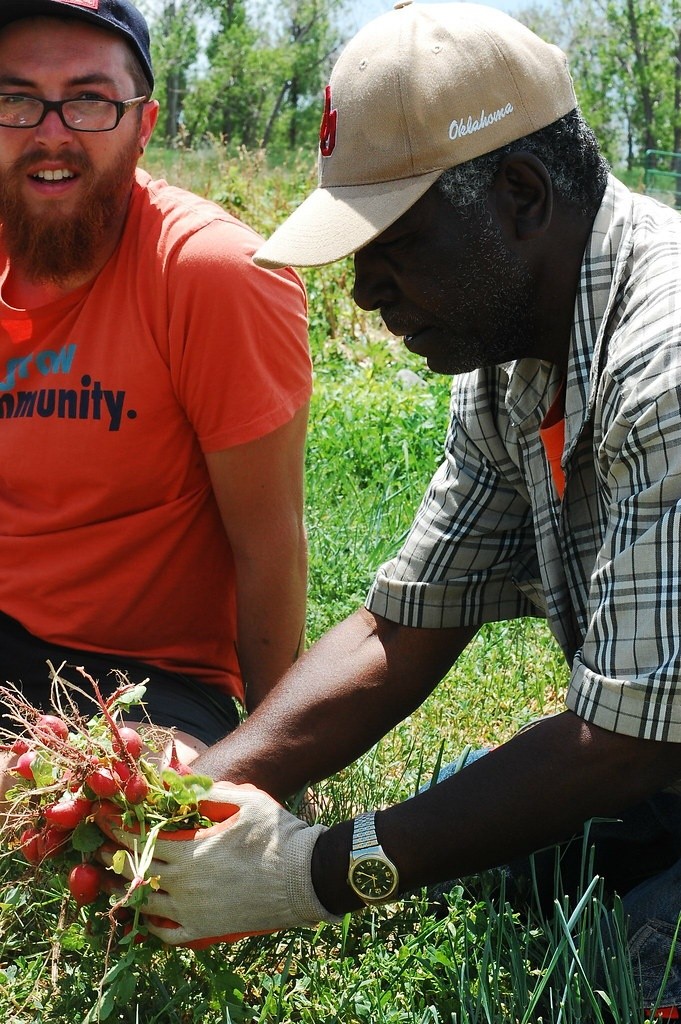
[348,810,400,905]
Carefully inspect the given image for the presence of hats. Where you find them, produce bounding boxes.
[0,0,156,100]
[251,2,578,271]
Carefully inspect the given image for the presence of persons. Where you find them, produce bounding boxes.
[0,0,311,775]
[94,0,681,1024]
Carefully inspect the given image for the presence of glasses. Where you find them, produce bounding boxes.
[1,93,147,131]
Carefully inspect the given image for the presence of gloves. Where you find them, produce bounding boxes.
[88,780,352,947]
